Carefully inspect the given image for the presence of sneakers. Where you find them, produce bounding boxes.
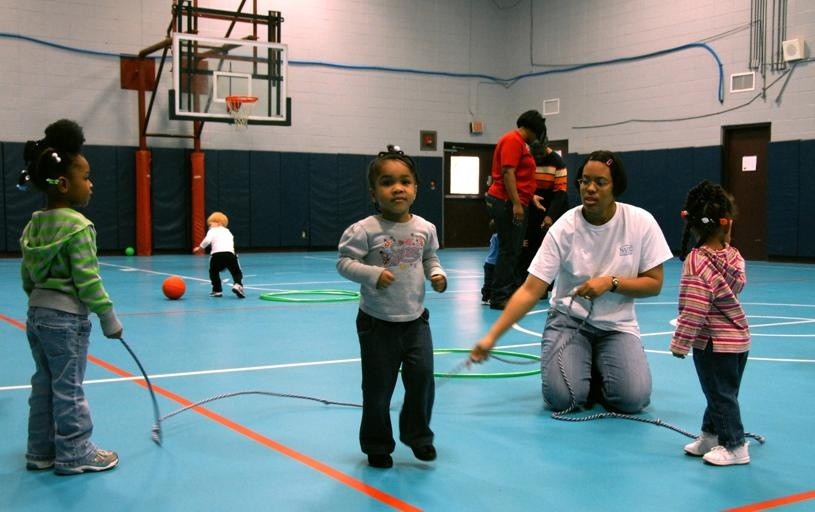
[208,291,223,298]
[703,439,752,465]
[231,282,247,300]
[361,452,394,469]
[24,457,58,471]
[683,432,721,455]
[412,440,438,463]
[57,447,118,476]
[490,299,508,310]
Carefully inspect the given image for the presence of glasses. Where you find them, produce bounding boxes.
[577,178,613,192]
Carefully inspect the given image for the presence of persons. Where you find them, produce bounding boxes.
[483,108,547,312]
[526,137,569,301]
[192,211,247,299]
[480,218,529,305]
[468,150,674,415]
[334,144,450,468]
[15,118,124,477]
[668,177,752,467]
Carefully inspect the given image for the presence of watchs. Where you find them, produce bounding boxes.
[610,276,620,293]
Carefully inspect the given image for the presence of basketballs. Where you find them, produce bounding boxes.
[162,276,187,299]
